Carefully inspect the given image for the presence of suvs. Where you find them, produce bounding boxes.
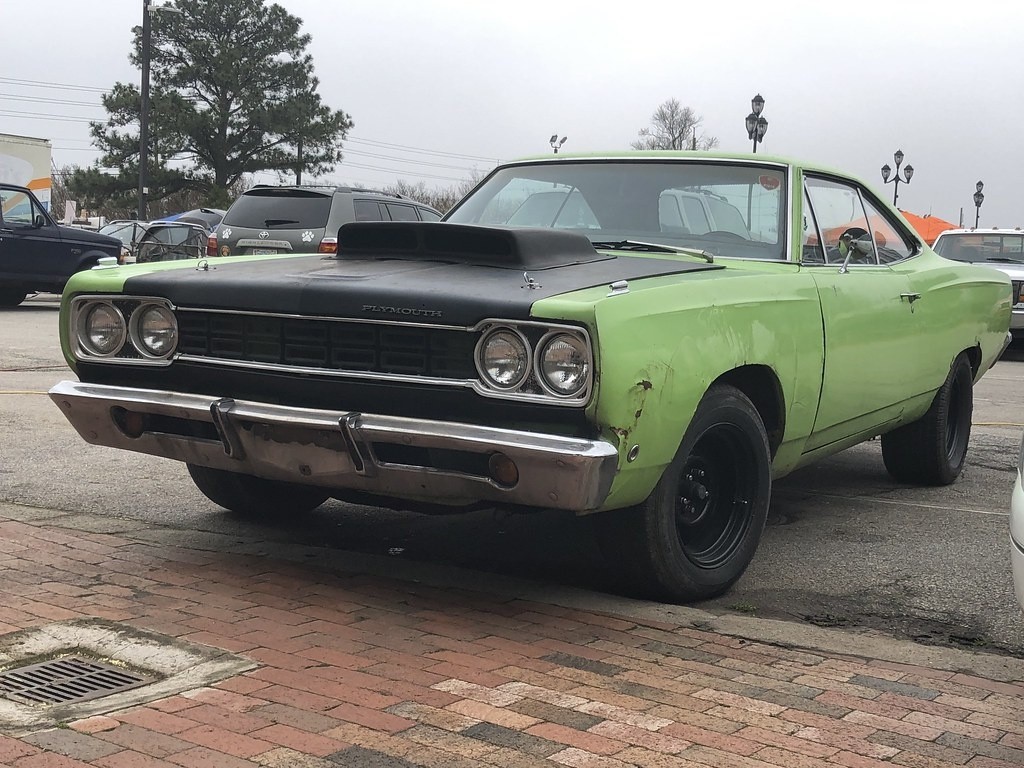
[203,184,447,257]
[0,182,125,311]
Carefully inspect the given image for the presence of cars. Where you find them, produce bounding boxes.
[50,147,1017,606]
[930,226,1024,334]
[94,219,151,257]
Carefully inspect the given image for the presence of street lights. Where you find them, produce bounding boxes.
[745,93,768,153]
[550,135,568,156]
[973,180,985,229]
[881,148,914,207]
